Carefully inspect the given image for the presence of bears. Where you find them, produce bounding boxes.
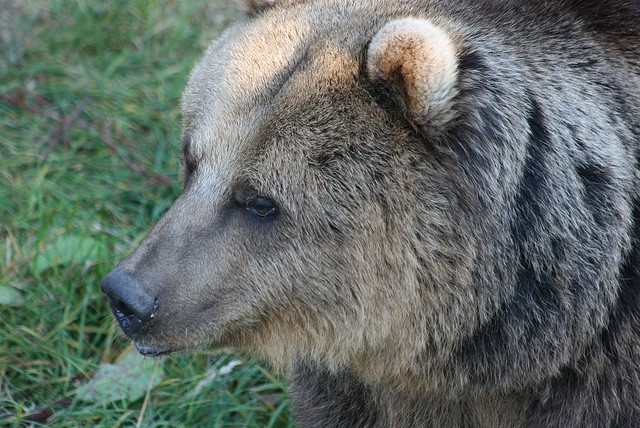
[100,1,640,428]
